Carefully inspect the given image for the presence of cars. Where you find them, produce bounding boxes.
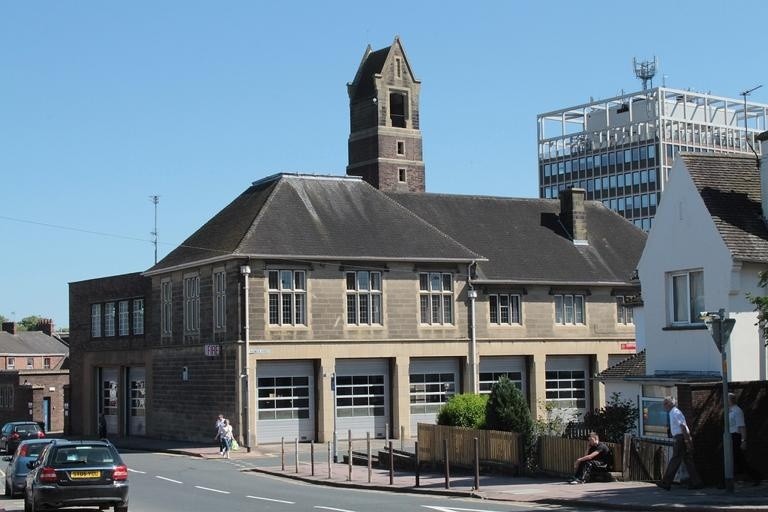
[0,421,44,456]
[24,438,130,512]
[2,439,69,499]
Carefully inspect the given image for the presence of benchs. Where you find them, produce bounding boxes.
[595,471,621,482]
[473,460,517,480]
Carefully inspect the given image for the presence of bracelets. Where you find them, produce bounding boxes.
[740,439,747,442]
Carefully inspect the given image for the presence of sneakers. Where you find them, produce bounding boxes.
[570,481,582,485]
[655,481,670,491]
[572,475,585,484]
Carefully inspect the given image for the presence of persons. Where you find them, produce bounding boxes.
[99,413,107,439]
[715,393,761,490]
[567,432,614,484]
[215,414,226,454]
[214,419,234,459]
[654,396,704,490]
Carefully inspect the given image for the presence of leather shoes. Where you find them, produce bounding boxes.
[752,473,764,486]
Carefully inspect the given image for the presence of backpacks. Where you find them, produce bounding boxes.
[601,447,615,470]
